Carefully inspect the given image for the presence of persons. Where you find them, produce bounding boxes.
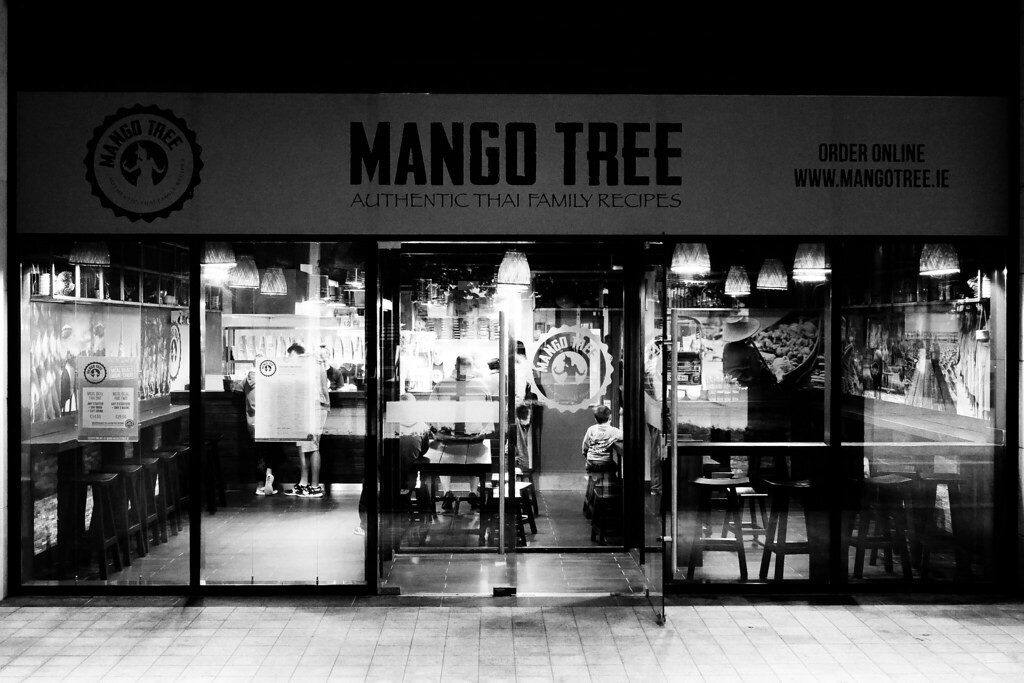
[720,317,778,391]
[581,404,624,491]
[231,345,357,501]
[391,340,540,499]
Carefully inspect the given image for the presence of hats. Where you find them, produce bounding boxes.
[450,356,480,381]
[595,406,612,418]
[722,316,760,342]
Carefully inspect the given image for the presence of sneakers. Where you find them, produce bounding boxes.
[256,486,278,495]
[284,484,324,498]
[265,475,274,496]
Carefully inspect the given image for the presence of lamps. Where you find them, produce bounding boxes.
[494,245,533,287]
[723,238,830,295]
[670,243,711,274]
[200,239,289,296]
[919,242,961,277]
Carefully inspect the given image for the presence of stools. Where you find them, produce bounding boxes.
[73,429,226,577]
[691,457,973,584]
